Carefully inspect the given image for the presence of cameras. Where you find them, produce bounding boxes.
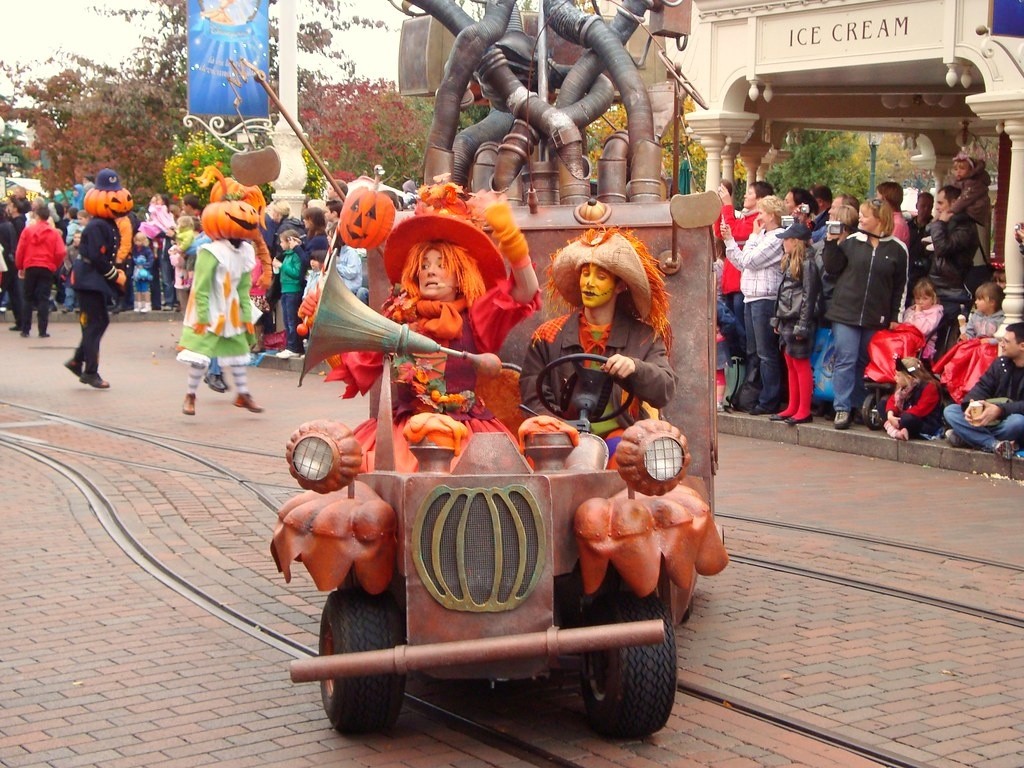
[825,222,845,235]
[376,167,385,174]
[800,204,809,213]
[780,216,798,228]
[1014,224,1022,232]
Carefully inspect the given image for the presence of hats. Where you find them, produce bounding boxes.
[548,226,671,340]
[384,182,508,295]
[775,224,812,240]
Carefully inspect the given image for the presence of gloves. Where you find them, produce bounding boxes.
[299,283,342,370]
[487,201,529,262]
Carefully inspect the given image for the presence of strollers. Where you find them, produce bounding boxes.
[861,302,964,430]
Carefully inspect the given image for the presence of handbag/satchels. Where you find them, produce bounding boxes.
[960,263,995,287]
[264,329,287,350]
[735,366,763,412]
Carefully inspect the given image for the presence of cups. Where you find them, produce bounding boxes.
[969,401,983,426]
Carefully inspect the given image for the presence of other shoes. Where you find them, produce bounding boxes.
[714,393,1020,462]
[0,297,182,337]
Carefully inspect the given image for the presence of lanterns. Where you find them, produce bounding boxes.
[341,186,396,250]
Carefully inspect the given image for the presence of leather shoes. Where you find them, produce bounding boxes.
[235,393,263,412]
[183,393,196,414]
[204,368,229,392]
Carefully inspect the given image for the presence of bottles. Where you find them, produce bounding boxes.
[273,257,279,274]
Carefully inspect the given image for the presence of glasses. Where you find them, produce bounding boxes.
[1001,337,1010,344]
[872,199,881,208]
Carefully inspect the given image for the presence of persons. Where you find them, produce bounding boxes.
[203,175,273,391]
[710,149,1024,459]
[108,193,203,317]
[251,163,417,361]
[518,227,680,471]
[175,200,265,414]
[64,168,135,387]
[298,190,538,470]
[0,175,96,336]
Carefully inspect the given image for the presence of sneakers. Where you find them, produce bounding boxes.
[276,349,305,359]
[80,371,110,387]
[65,358,83,376]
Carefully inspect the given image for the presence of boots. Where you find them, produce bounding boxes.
[251,325,263,353]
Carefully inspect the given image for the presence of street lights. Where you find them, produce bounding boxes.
[870,131,883,200]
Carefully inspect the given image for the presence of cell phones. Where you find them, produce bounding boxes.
[721,214,726,228]
